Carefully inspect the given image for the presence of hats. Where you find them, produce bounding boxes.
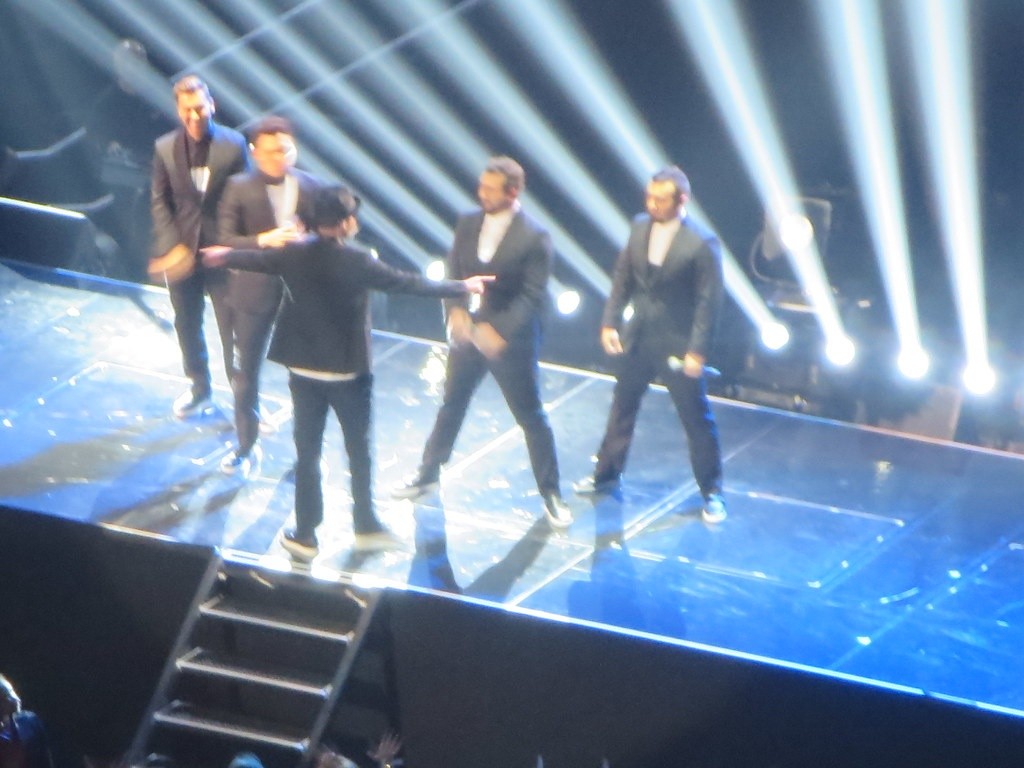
[300,186,361,223]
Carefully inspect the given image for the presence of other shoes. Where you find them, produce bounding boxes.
[356,530,414,556]
[701,493,728,522]
[389,469,441,497]
[280,526,320,563]
[219,450,258,474]
[571,474,623,493]
[171,389,214,418]
[542,493,574,528]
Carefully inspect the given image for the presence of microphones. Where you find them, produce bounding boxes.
[668,357,722,378]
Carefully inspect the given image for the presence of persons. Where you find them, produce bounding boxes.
[151,76,249,417]
[197,116,496,558]
[390,155,572,529]
[572,167,729,523]
